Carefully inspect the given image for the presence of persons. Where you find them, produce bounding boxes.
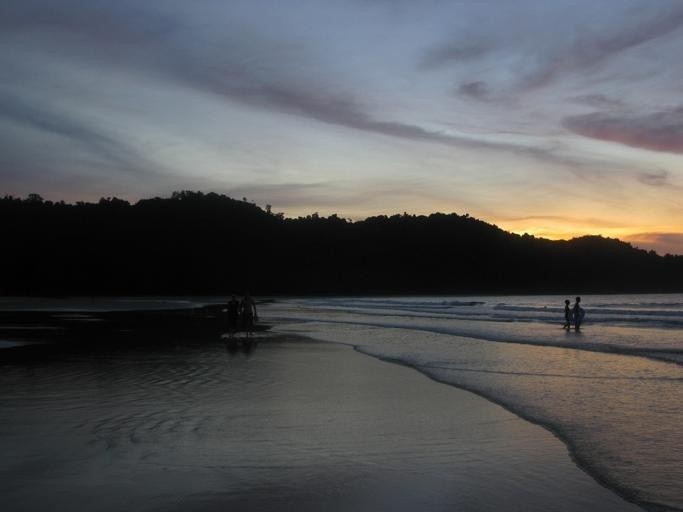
[226,293,239,342]
[239,291,257,339]
[572,296,585,333]
[562,299,572,329]
